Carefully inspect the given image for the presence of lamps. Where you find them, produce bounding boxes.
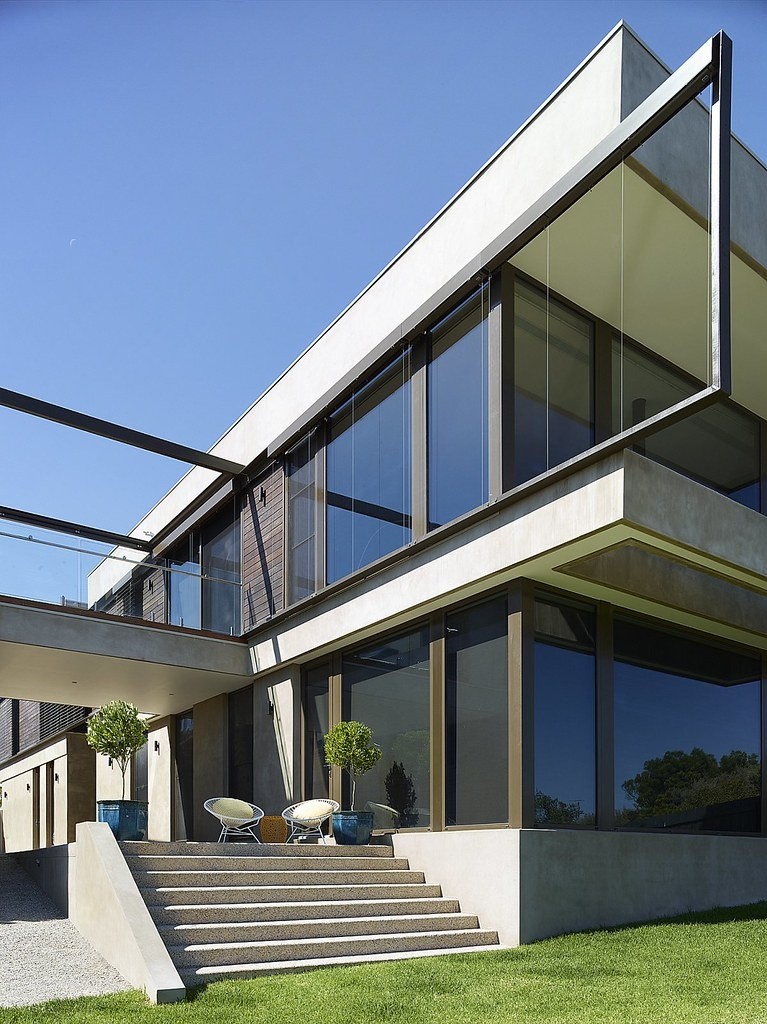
[147,579,153,591]
[266,700,274,715]
[258,486,265,501]
[27,784,30,790]
[154,740,159,752]
[109,757,113,766]
[55,773,59,781]
[4,792,7,798]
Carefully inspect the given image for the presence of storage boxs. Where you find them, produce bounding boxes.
[261,816,287,843]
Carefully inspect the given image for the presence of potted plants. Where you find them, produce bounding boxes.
[87,700,149,842]
[324,722,376,845]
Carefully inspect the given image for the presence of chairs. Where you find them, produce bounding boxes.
[204,798,264,844]
[280,798,339,844]
